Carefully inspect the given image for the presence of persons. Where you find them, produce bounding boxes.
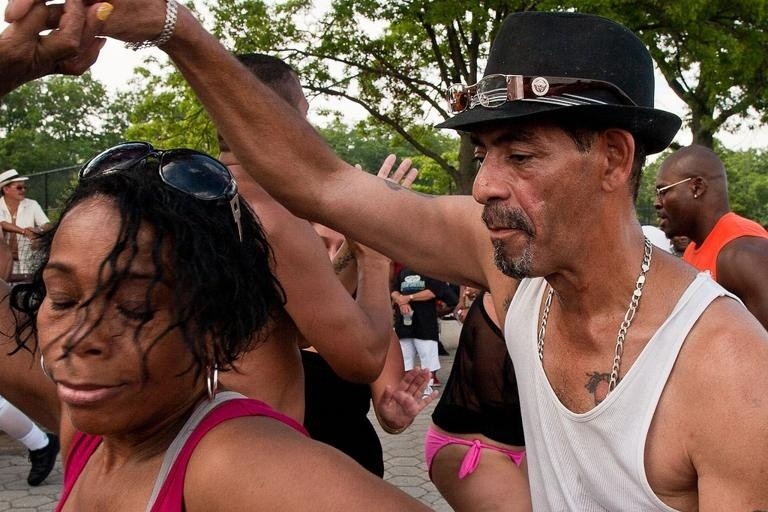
[0,1,768,511]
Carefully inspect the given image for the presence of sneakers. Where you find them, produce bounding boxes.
[431,371,440,387]
[27,431,60,487]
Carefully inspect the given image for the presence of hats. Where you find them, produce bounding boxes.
[0,168,30,191]
[435,9,681,156]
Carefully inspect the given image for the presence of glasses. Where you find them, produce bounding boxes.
[77,140,247,244]
[10,184,27,190]
[654,176,693,198]
[446,73,641,116]
